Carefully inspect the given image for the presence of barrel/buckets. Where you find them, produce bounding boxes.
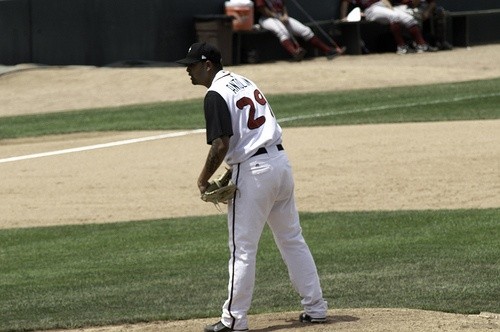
[194,15,233,66]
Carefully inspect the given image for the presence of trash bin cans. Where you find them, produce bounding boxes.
[193,13,234,67]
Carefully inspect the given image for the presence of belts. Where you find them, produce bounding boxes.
[251,144,284,157]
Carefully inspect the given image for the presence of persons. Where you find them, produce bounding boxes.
[177,42,328,332]
[399,0,453,52]
[256,0,346,61]
[340,0,427,55]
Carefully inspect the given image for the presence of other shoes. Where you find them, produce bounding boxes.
[293,48,303,58]
[327,45,347,61]
[300,312,326,323]
[204,321,249,332]
[396,42,453,56]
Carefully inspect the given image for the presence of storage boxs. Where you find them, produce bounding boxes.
[192,14,234,66]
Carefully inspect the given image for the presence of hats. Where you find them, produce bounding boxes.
[178,43,222,64]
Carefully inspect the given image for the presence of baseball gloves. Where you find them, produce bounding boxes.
[200,180,241,213]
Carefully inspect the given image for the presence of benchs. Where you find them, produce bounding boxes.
[230,8,500,64]
